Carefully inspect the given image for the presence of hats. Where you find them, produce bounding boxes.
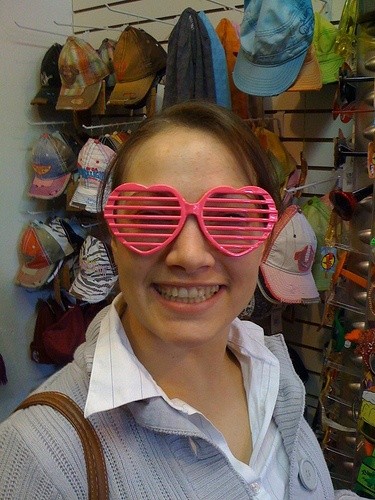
[15,129,134,365]
[31,27,168,111]
[253,122,341,307]
[232,0,345,93]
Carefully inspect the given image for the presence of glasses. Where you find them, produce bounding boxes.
[346,9,375,36]
[339,69,375,91]
[331,96,375,123]
[104,182,279,258]
[311,127,375,500]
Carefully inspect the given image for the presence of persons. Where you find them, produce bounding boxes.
[0,101,373,500]
